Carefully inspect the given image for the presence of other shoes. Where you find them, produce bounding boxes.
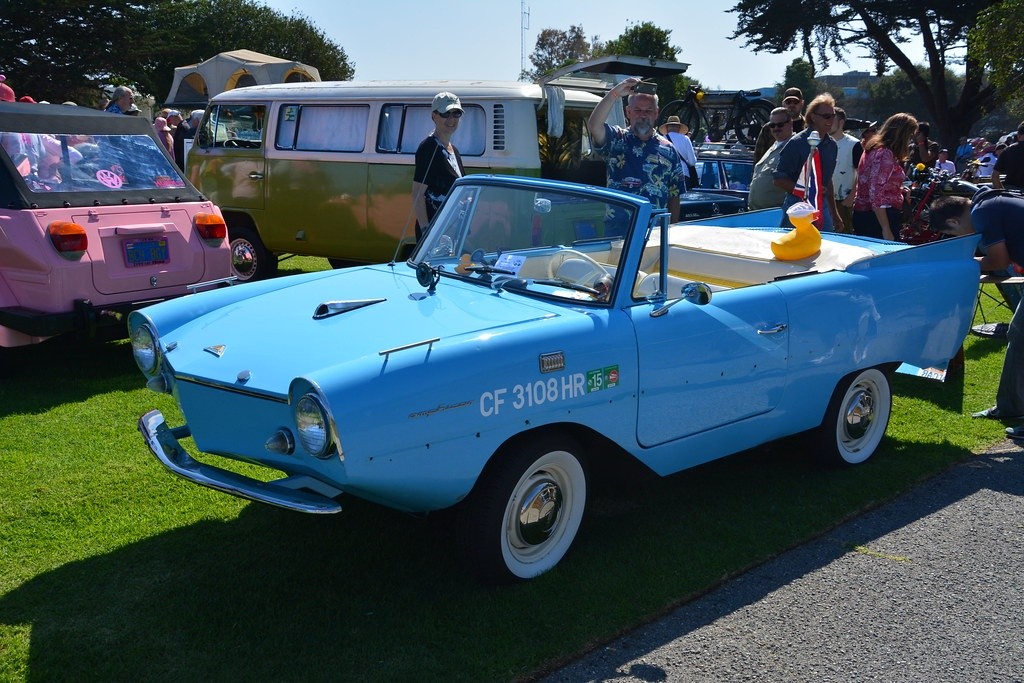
[972,405,1024,420]
[1006,425,1024,439]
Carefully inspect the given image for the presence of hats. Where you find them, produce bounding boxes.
[659,116,688,135]
[783,88,803,102]
[63,101,78,106]
[152,117,172,131]
[859,120,877,139]
[432,92,465,116]
[127,104,142,112]
[166,110,180,119]
[19,96,37,103]
[0,81,16,102]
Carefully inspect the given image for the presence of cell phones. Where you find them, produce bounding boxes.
[634,81,657,96]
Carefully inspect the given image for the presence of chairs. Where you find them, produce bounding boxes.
[637,272,732,303]
[968,274,1011,335]
[556,256,648,291]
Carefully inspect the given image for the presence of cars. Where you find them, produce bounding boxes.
[0,102,233,357]
[680,149,757,220]
[127,170,984,582]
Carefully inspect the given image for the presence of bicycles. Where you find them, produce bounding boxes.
[659,85,777,147]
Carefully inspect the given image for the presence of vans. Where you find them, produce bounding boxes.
[186,54,706,281]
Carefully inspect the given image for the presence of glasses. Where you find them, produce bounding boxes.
[434,110,462,119]
[785,99,801,104]
[768,119,792,129]
[814,112,836,119]
[870,128,878,135]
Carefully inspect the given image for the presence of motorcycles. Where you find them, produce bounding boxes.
[901,160,994,244]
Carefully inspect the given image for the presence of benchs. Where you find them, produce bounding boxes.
[658,246,808,290]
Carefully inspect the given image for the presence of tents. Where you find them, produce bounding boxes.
[160,45,322,106]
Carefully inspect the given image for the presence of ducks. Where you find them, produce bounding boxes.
[770,202,822,260]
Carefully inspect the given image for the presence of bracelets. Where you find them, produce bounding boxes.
[918,141,924,145]
[611,89,618,99]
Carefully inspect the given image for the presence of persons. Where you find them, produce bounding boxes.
[828,105,864,235]
[991,120,1024,190]
[412,91,468,247]
[929,188,1024,440]
[906,121,940,170]
[773,91,844,234]
[937,147,956,176]
[658,115,698,191]
[587,76,687,238]
[853,111,918,243]
[0,74,206,192]
[956,131,1018,179]
[753,86,805,173]
[746,106,798,210]
